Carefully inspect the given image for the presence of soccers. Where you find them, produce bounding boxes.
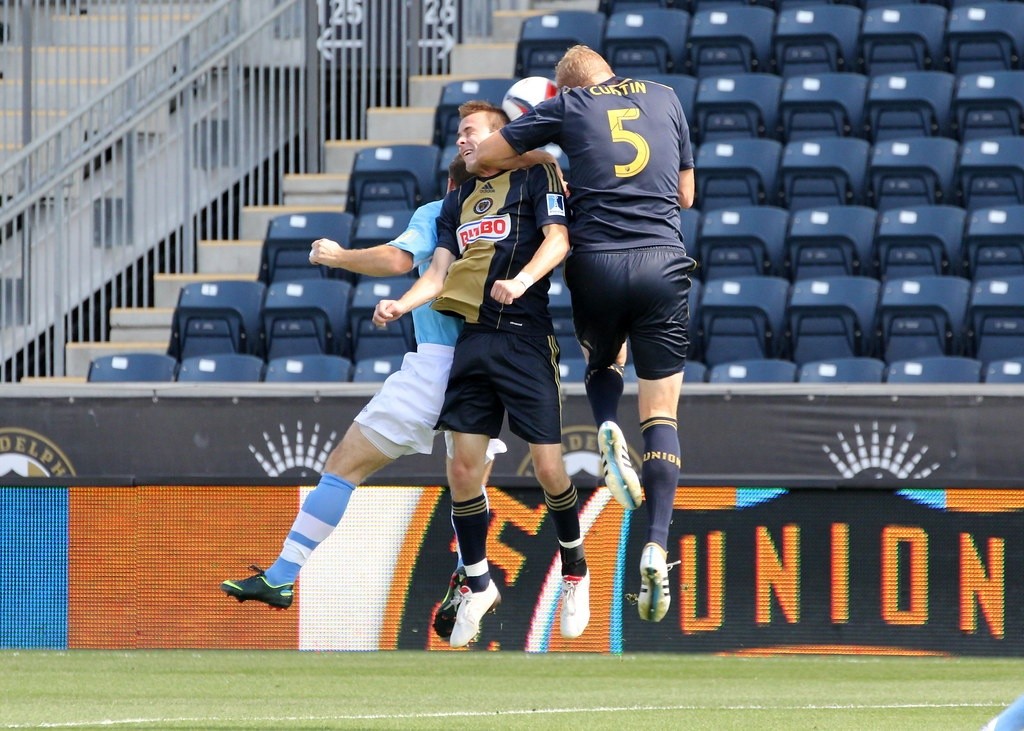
[498,74,560,123]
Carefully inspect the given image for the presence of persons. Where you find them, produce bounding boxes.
[371,99,590,649]
[219,153,475,640]
[475,44,698,622]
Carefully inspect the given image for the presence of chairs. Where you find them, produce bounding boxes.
[84,0,1024,388]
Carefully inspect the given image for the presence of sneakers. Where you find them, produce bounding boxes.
[450,579,503,650]
[434,567,470,638]
[637,542,672,625]
[561,567,590,638]
[221,575,294,612]
[597,420,642,512]
